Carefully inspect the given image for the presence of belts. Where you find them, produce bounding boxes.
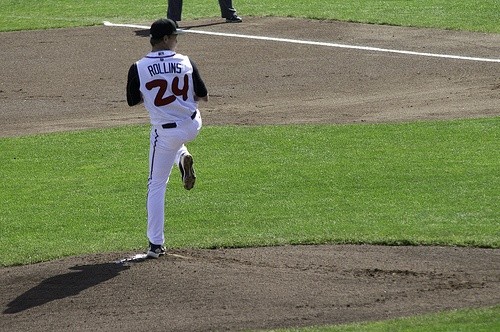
[162,110,197,128]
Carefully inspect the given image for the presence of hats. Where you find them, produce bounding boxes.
[151,18,183,35]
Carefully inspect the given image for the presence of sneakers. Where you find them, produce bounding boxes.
[179,153,196,190]
[147,241,166,259]
[226,15,243,23]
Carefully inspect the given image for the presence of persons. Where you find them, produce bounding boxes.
[126,19,209,259]
[167,0,242,27]
